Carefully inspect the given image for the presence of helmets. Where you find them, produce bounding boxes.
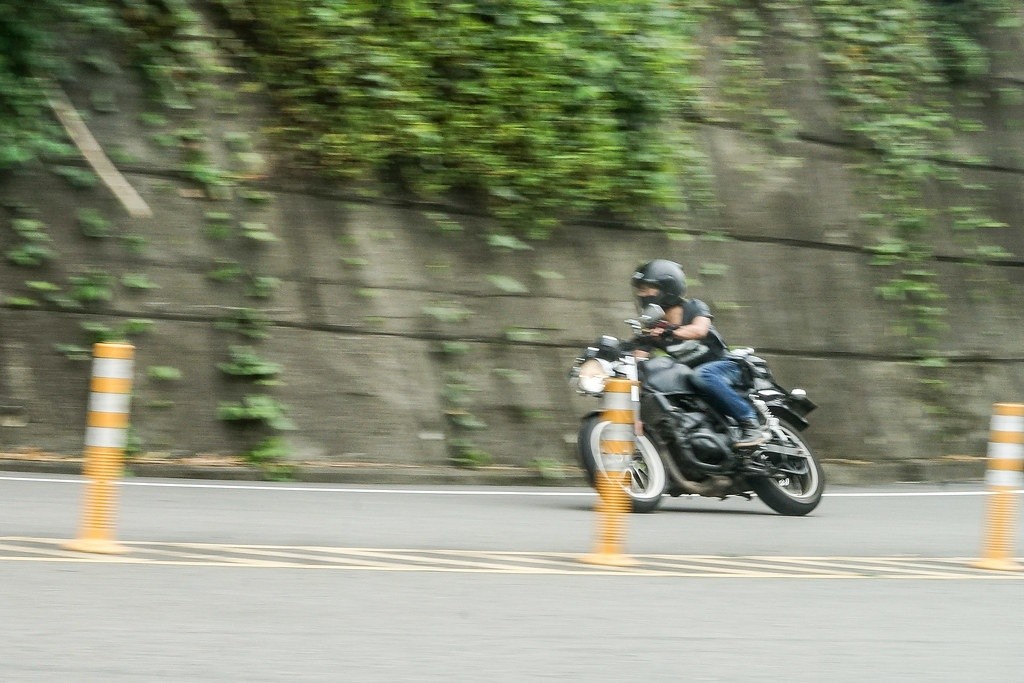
[629,259,685,310]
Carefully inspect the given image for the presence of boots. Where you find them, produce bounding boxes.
[732,418,767,452]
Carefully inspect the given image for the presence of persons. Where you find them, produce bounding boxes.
[597,259,772,449]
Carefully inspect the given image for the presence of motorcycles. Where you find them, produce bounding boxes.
[567,304,824,518]
